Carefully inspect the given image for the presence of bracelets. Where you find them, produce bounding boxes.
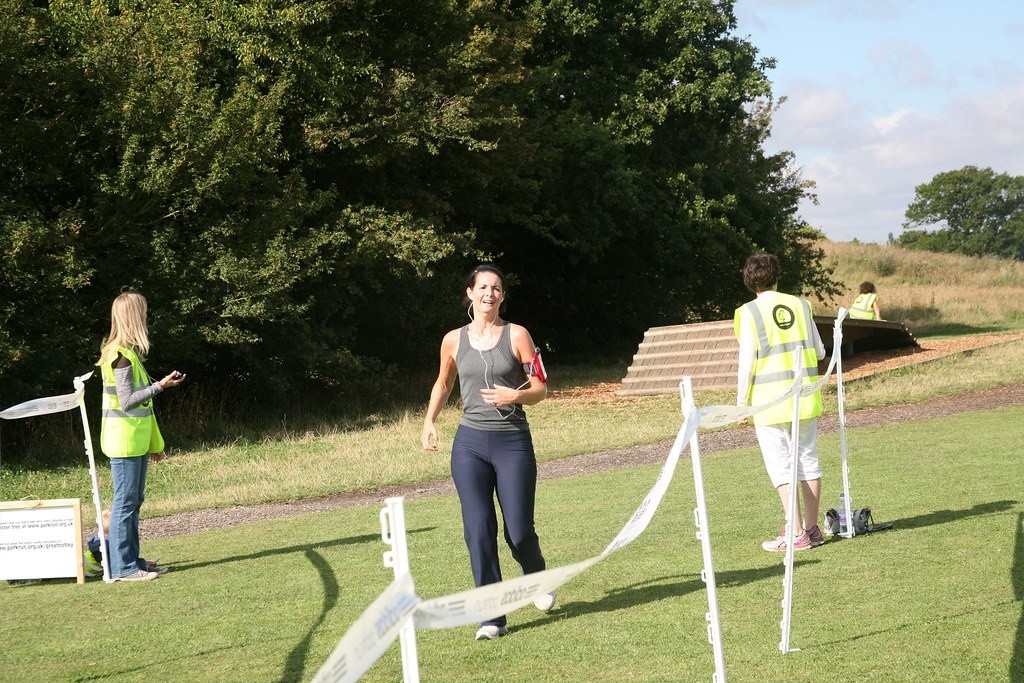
[156,381,164,389]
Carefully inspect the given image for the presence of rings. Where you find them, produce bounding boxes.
[490,400,496,406]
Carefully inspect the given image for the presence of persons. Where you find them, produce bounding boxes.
[732,251,828,555]
[848,281,886,321]
[419,265,556,642]
[95,292,186,583]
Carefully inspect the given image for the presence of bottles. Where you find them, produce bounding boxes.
[839,493,850,538]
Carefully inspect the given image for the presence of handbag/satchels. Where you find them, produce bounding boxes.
[823,508,893,536]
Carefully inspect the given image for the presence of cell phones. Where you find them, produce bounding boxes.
[532,352,549,382]
[173,373,182,380]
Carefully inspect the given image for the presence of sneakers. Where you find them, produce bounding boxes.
[106,569,158,584]
[474,624,508,640]
[776,526,824,546]
[761,531,812,551]
[142,564,168,574]
[519,565,556,611]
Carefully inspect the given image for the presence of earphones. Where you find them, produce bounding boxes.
[500,298,504,304]
